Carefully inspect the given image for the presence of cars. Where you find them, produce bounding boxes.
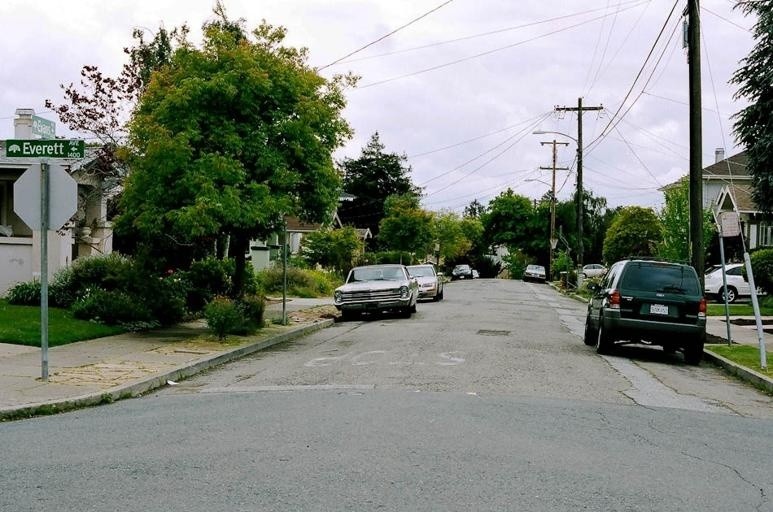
[472,269,480,278]
[573,264,609,279]
[397,266,445,301]
[334,264,422,319]
[522,264,546,283]
[451,264,472,279]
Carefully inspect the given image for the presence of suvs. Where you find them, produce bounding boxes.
[583,256,705,367]
[702,261,766,303]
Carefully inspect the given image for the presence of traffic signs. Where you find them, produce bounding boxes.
[5,139,86,162]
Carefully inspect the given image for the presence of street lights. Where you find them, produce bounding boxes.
[531,129,585,270]
[524,178,558,282]
